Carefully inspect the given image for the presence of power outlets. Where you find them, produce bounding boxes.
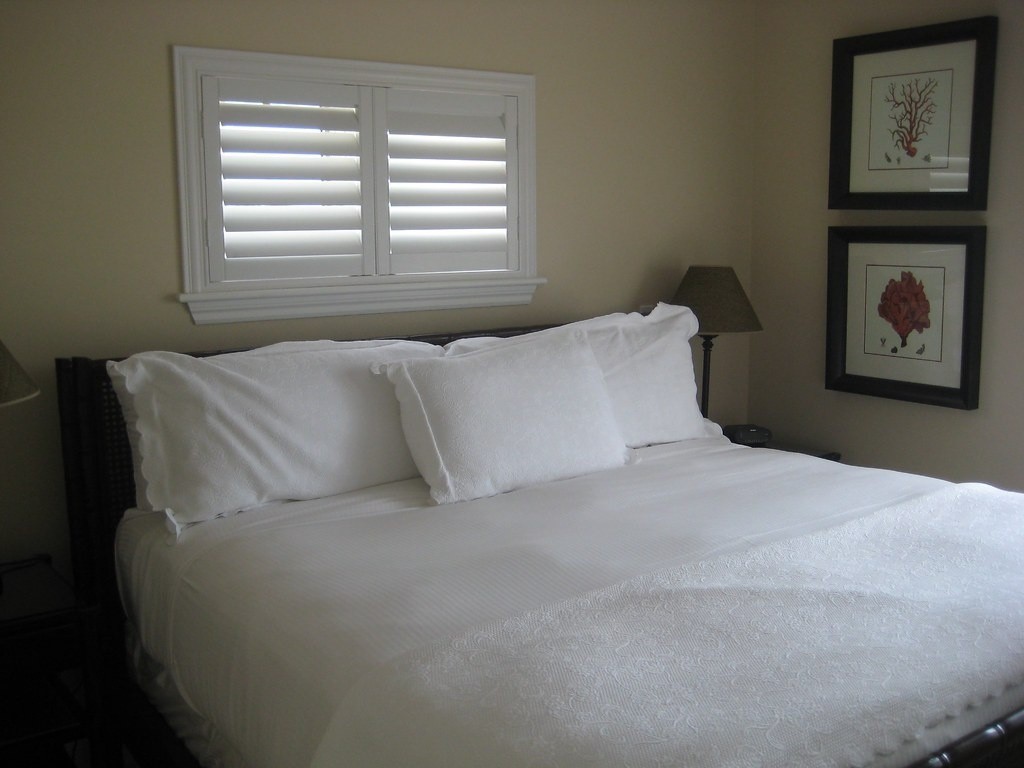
[639,305,656,315]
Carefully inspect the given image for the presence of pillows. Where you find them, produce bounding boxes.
[105,340,446,547]
[443,302,723,448]
[371,328,630,507]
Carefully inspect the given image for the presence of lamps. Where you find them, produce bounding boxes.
[0,340,41,408]
[670,265,764,418]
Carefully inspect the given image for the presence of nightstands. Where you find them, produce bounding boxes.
[750,440,841,462]
[53,322,1024,768]
[0,551,109,768]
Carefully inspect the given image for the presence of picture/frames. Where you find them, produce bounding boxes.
[827,14,1000,213]
[825,225,988,411]
